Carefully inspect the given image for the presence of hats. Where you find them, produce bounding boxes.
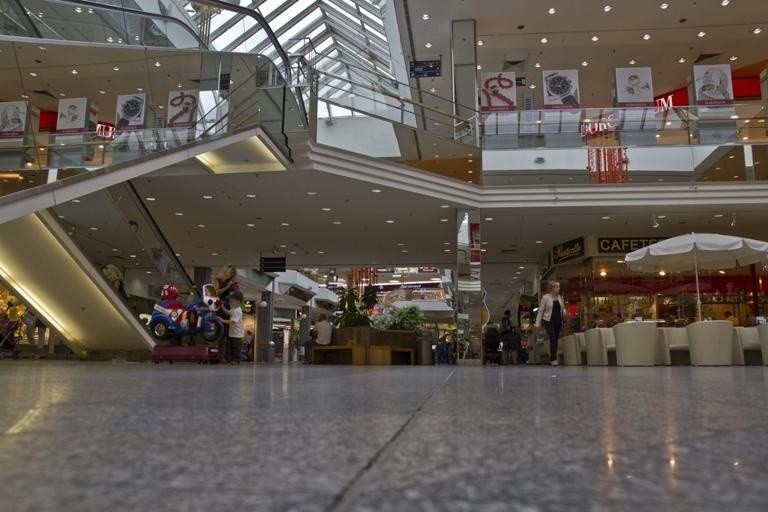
[226,291,243,300]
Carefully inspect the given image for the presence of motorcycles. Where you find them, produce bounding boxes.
[149,283,225,343]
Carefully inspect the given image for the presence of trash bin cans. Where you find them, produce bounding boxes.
[437,333,455,365]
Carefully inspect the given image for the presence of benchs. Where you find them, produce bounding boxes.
[311,343,367,364]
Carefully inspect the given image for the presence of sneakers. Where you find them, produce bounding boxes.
[231,359,240,365]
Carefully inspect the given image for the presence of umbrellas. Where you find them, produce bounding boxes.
[624,231,768,321]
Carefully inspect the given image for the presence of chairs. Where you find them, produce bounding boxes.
[535,319,768,366]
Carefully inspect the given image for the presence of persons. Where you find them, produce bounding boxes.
[10,296,47,359]
[663,311,675,327]
[500,310,520,365]
[217,291,243,365]
[483,320,499,351]
[0,312,10,343]
[534,281,567,367]
[102,264,129,300]
[725,312,739,326]
[143,241,177,284]
[129,220,139,234]
[246,330,252,361]
[215,261,239,363]
[303,314,332,365]
[431,330,470,364]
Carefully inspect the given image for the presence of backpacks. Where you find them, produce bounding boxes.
[502,315,512,333]
